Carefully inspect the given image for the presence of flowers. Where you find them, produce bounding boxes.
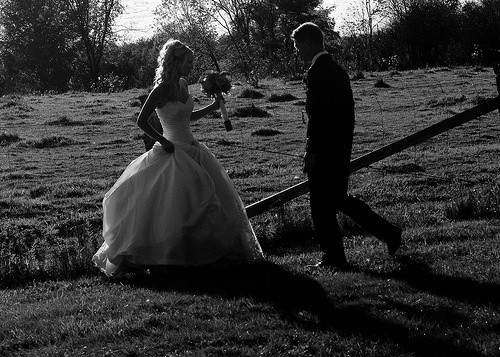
[197,70,233,132]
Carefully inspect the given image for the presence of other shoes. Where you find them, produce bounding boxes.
[304,261,347,270]
[384,223,403,255]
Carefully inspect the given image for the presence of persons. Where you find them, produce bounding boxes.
[292,21,401,269]
[92,39,264,277]
